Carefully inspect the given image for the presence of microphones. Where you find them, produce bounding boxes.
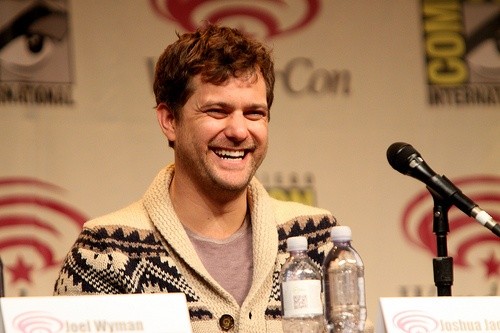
[387,141,500,237]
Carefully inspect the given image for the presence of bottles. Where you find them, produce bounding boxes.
[280,233,327,332]
[323,226,367,332]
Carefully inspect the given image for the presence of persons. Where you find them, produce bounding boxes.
[53,20,374,333]
